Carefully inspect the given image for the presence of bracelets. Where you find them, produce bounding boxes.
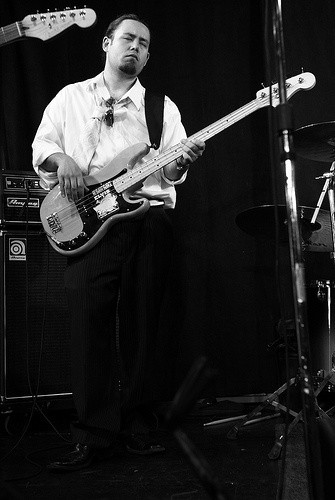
[176,159,188,170]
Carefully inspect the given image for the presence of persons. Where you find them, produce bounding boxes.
[31,13,205,467]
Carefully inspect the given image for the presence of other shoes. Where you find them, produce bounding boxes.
[86,427,115,460]
[125,424,165,452]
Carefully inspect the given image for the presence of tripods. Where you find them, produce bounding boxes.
[202,0,335,460]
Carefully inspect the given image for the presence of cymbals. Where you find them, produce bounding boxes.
[277,120,335,163]
[236,203,335,253]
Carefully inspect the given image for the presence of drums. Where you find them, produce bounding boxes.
[307,278,335,394]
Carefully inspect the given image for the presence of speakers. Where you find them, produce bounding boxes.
[0,230,125,414]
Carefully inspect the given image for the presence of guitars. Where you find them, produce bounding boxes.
[0,5,96,44]
[38,68,316,257]
